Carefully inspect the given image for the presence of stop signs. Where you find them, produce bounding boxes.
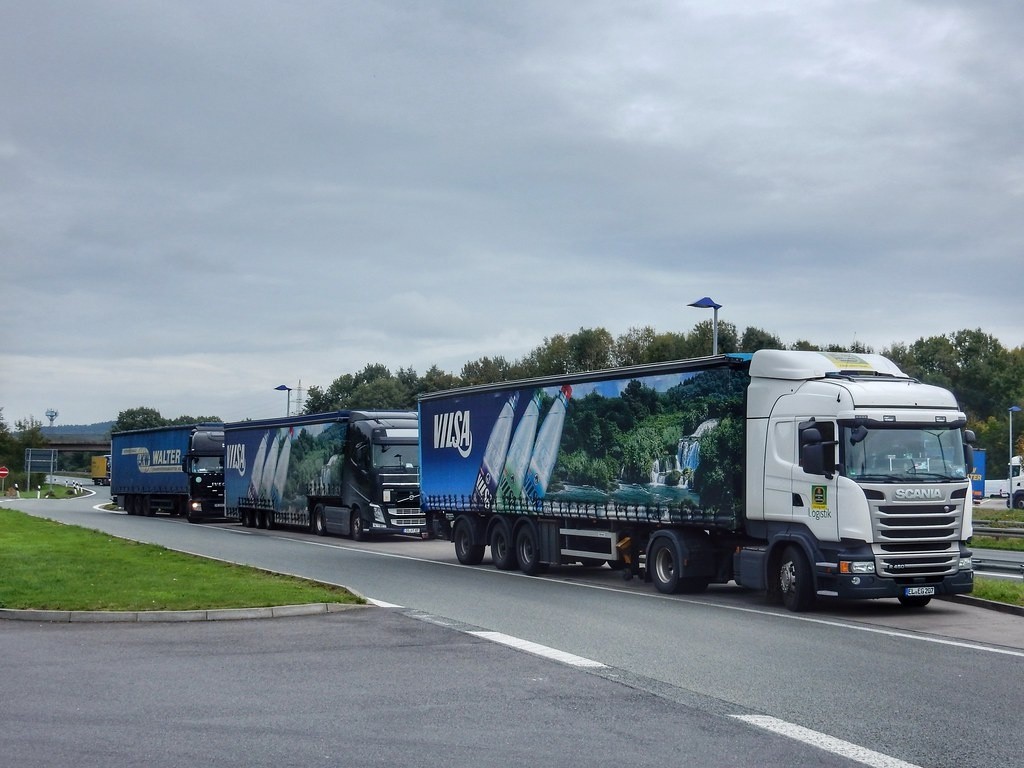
[0,467,9,479]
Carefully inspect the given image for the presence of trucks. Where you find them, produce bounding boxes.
[1004,455,1024,510]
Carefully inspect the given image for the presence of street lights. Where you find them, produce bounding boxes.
[274,385,293,416]
[688,296,721,357]
[1007,406,1021,510]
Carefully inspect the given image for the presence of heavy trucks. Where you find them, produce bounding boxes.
[109,421,228,525]
[222,410,441,542]
[91,455,112,486]
[415,349,981,612]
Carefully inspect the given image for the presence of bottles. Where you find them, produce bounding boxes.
[270,427,294,511]
[472,391,519,510]
[516,385,572,516]
[256,428,282,509]
[246,429,271,509]
[492,388,543,513]
